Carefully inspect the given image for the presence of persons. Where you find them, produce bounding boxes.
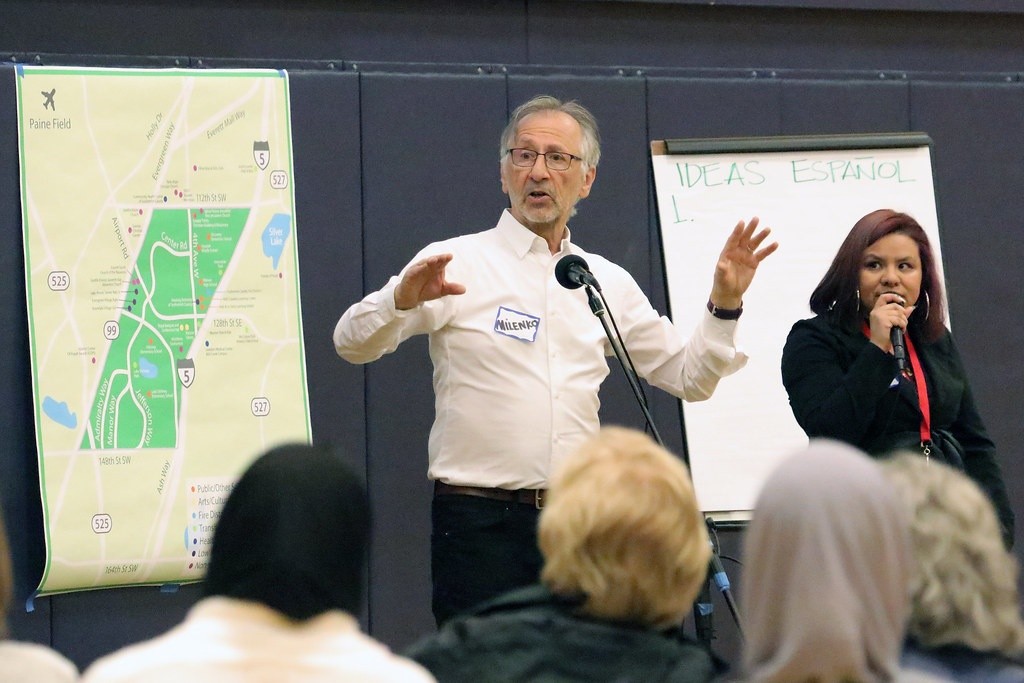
[877,449,1024,681]
[0,509,80,683]
[739,440,948,682]
[331,93,781,636]
[83,444,440,683]
[397,427,740,683]
[781,206,1017,550]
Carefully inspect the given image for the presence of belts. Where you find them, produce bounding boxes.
[436,481,549,510]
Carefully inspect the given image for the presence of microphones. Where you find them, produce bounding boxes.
[555,254,600,291]
[888,301,905,370]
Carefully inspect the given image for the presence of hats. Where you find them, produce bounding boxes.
[205,447,371,606]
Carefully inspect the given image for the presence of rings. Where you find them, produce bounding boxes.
[747,245,753,253]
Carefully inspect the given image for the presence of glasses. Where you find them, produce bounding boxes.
[505,147,582,171]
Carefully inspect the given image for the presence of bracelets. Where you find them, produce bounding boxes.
[707,299,743,320]
